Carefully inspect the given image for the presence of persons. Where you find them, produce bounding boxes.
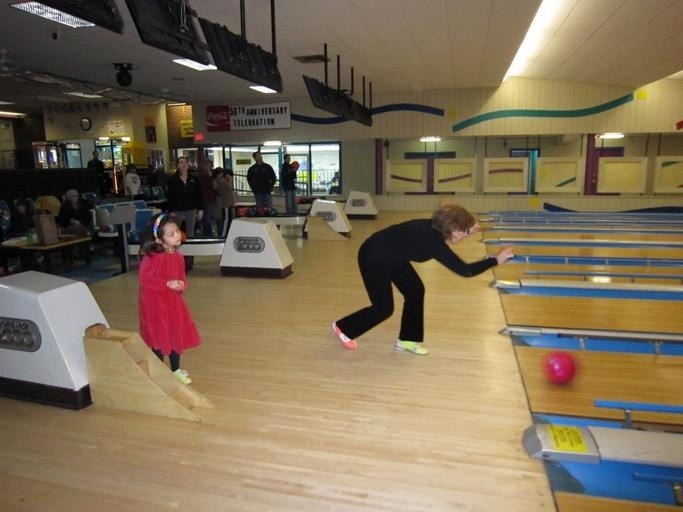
[134,213,200,386]
[122,162,140,197]
[61,188,92,234]
[164,147,300,240]
[85,150,104,170]
[330,203,517,359]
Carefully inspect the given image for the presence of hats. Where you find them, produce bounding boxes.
[66,190,79,199]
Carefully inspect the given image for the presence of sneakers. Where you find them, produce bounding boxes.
[174,369,192,384]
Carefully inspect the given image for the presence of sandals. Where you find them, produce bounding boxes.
[395,339,429,355]
[332,321,356,348]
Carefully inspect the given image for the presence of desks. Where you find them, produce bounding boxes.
[0,233,92,274]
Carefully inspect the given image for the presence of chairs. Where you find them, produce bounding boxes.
[94,200,154,242]
